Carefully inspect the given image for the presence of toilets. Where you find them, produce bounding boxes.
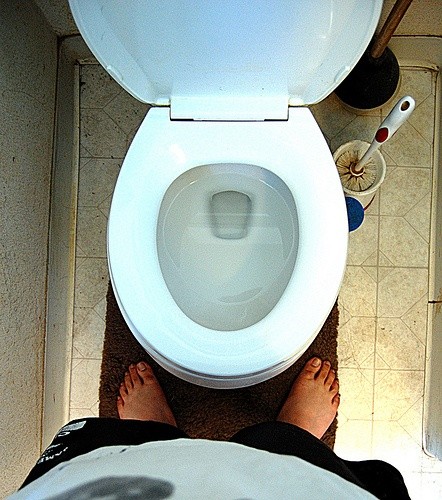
[68,0,384,390]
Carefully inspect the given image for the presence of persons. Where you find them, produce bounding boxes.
[2,355,409,499]
